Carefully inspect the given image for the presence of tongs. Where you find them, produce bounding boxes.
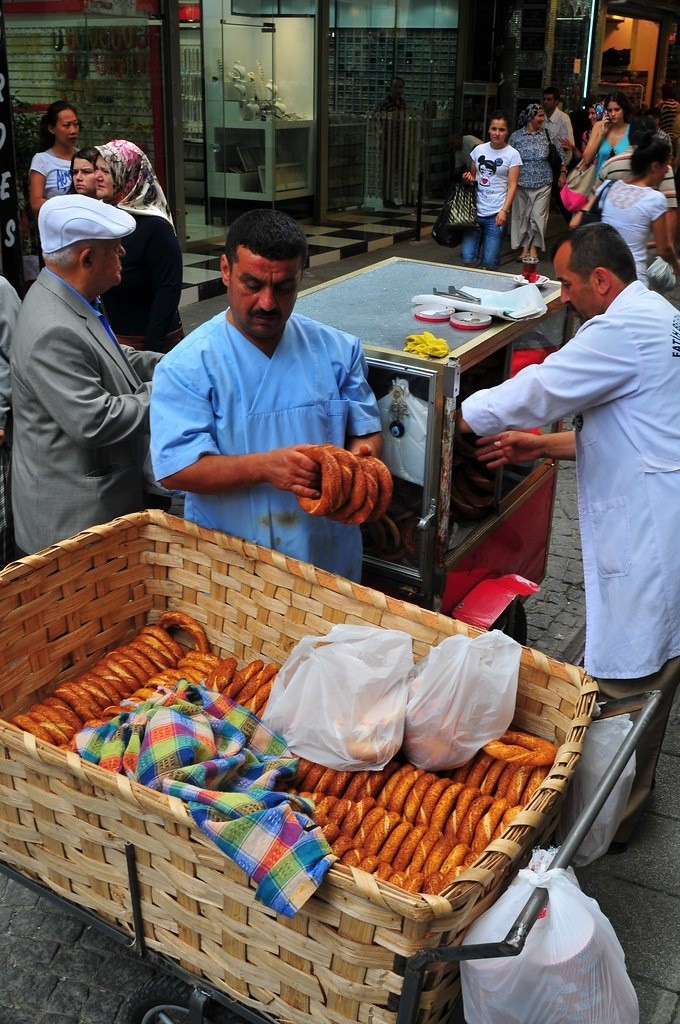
[433,286,481,305]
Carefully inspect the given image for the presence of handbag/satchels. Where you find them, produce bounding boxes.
[560,700,641,865]
[578,178,618,228]
[545,128,562,184]
[405,628,522,769]
[645,257,677,292]
[561,159,599,212]
[432,179,479,248]
[263,624,415,769]
[457,861,640,1024]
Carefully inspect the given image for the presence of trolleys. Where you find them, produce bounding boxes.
[290,254,571,647]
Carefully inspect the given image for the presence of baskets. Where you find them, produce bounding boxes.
[0,507,601,1023]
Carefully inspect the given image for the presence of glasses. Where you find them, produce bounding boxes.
[607,149,615,160]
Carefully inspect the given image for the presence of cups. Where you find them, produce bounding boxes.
[523,257,539,283]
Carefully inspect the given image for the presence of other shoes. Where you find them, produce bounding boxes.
[517,255,528,262]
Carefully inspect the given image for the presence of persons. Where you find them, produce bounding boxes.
[568,135,680,292]
[0,275,20,570]
[27,99,83,216]
[540,85,680,311]
[446,125,485,177]
[508,104,571,262]
[92,138,186,355]
[12,196,167,555]
[458,113,524,271]
[145,209,382,583]
[454,223,680,826]
[64,146,99,199]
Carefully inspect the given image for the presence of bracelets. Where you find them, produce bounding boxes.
[560,170,567,174]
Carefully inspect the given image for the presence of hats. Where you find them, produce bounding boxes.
[38,194,136,253]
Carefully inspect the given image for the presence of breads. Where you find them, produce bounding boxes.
[294,443,392,524]
[10,611,557,899]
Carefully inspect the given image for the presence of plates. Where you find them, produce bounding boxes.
[513,275,549,288]
